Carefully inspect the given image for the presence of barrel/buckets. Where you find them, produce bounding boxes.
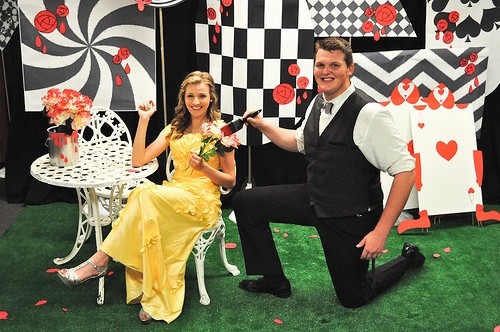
[45,127,80,168]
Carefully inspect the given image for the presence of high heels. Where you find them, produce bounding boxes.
[139,308,151,321]
[57,259,108,288]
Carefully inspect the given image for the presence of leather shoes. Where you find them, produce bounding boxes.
[402,241,426,266]
[238,277,291,298]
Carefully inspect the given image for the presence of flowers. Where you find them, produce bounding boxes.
[40,87,94,131]
[185,121,240,175]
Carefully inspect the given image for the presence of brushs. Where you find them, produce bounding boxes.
[220,109,262,136]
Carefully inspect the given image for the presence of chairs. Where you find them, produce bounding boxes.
[166,150,240,306]
[77,105,156,244]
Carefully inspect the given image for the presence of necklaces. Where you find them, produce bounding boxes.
[189,117,206,132]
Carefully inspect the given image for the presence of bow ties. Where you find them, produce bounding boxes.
[314,95,334,114]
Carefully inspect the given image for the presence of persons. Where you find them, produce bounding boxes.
[232,37,425,309]
[57,71,236,323]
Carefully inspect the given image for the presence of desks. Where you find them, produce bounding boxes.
[30,144,159,305]
